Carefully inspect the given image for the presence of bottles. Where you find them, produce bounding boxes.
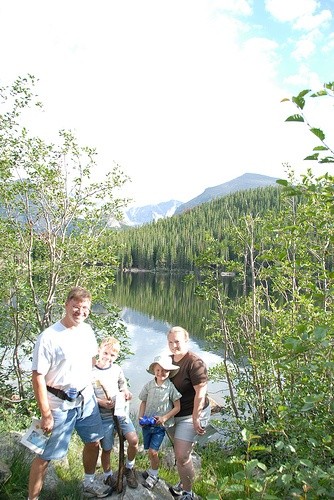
[66,387,78,410]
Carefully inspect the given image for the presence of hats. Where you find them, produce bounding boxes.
[146,355,181,378]
[208,396,222,415]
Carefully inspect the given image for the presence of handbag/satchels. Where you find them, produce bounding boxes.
[46,384,96,418]
[198,394,212,429]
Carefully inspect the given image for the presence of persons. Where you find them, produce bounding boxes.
[138,355,183,491]
[167,326,212,500]
[93,336,139,495]
[27,286,106,500]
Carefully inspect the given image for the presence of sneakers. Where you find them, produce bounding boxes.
[103,474,119,490]
[83,476,111,498]
[169,486,194,500]
[124,465,138,488]
[141,465,160,489]
